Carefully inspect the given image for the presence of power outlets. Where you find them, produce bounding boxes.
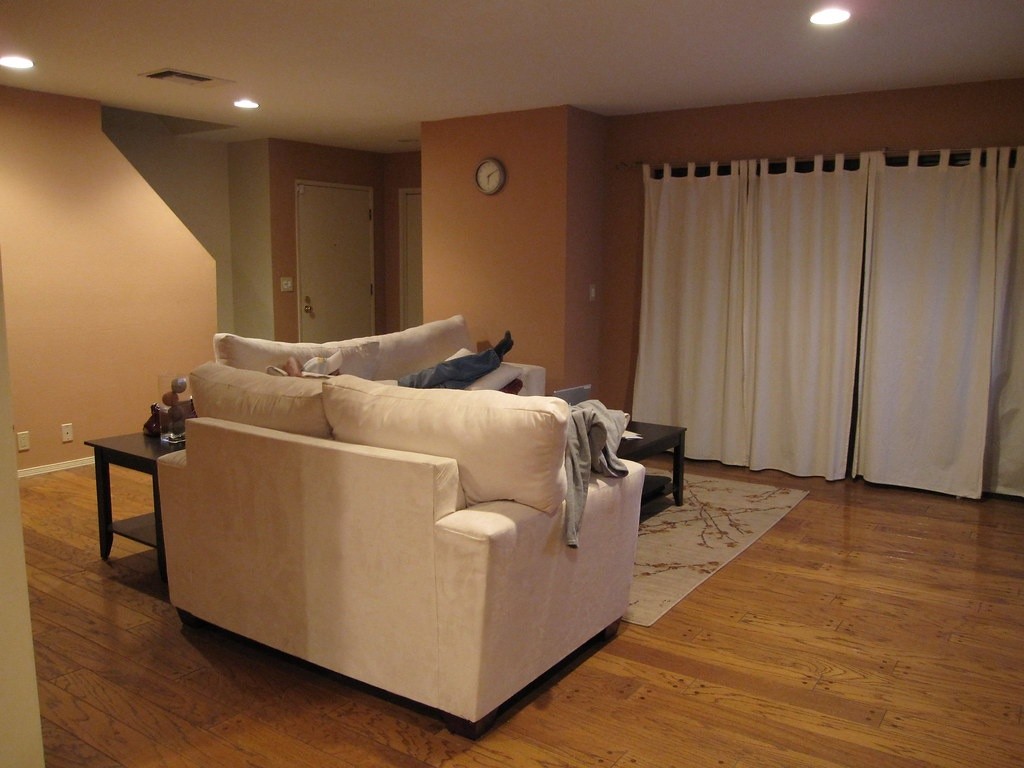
[61,423,73,443]
[17,431,29,451]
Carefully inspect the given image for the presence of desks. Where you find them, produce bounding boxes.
[84,431,185,582]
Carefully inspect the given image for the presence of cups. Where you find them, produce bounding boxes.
[156,373,190,445]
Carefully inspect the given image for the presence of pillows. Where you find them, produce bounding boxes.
[322,374,570,515]
[188,360,334,441]
[213,315,467,382]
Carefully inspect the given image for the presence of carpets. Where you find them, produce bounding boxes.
[622,467,808,627]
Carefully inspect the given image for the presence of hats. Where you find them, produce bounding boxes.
[301,349,343,375]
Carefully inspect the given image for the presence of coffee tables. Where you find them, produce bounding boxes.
[616,421,687,506]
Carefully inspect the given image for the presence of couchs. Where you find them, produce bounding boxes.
[446,348,547,397]
[155,417,644,728]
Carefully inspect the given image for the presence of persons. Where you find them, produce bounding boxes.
[287,331,513,389]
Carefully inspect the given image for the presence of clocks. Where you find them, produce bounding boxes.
[475,157,506,195]
